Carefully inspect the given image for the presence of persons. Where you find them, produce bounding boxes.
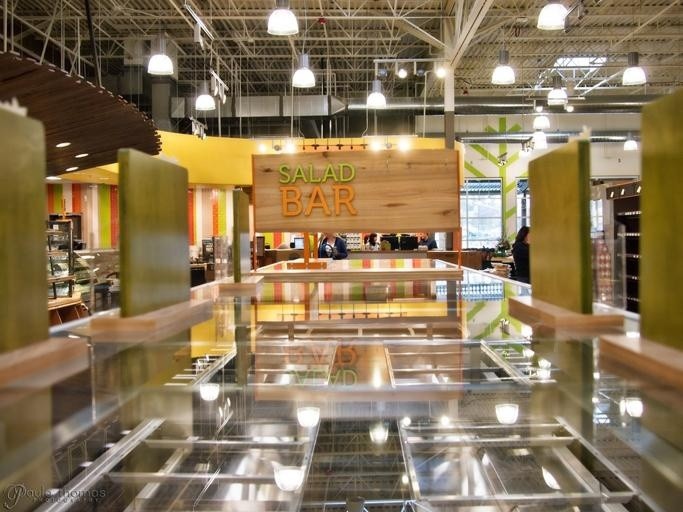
[317,232,350,260]
[366,233,382,251]
[417,232,437,251]
[511,225,532,284]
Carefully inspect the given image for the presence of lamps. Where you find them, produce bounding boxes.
[147,1,648,112]
[189,115,207,141]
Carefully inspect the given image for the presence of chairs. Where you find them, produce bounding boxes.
[92,271,120,313]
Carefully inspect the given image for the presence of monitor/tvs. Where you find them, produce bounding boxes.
[202,240,220,264]
[294,238,304,249]
[381,237,399,250]
[400,236,418,250]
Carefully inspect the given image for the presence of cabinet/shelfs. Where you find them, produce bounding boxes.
[0,257,683,512]
[44,218,76,301]
[605,180,642,313]
